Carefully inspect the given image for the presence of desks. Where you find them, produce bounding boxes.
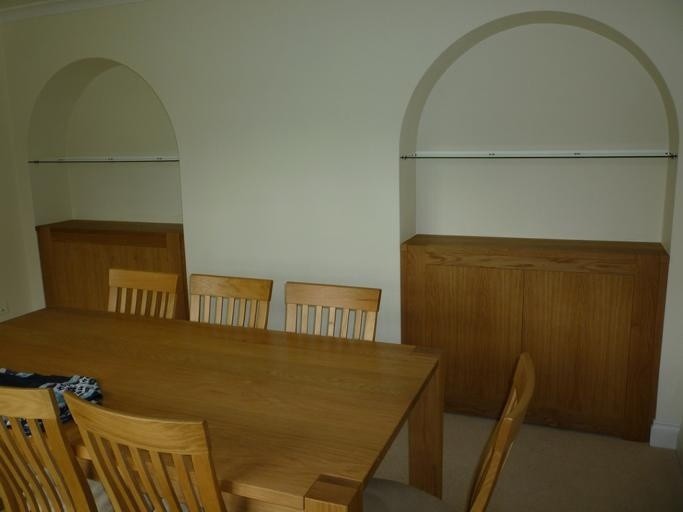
[0,302,449,512]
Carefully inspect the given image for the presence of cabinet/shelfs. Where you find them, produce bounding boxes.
[35,219,189,320]
[402,235,671,445]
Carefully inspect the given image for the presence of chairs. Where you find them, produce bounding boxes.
[105,271,182,319]
[186,272,275,331]
[280,279,384,344]
[353,351,537,512]
[59,386,230,512]
[0,386,100,511]
